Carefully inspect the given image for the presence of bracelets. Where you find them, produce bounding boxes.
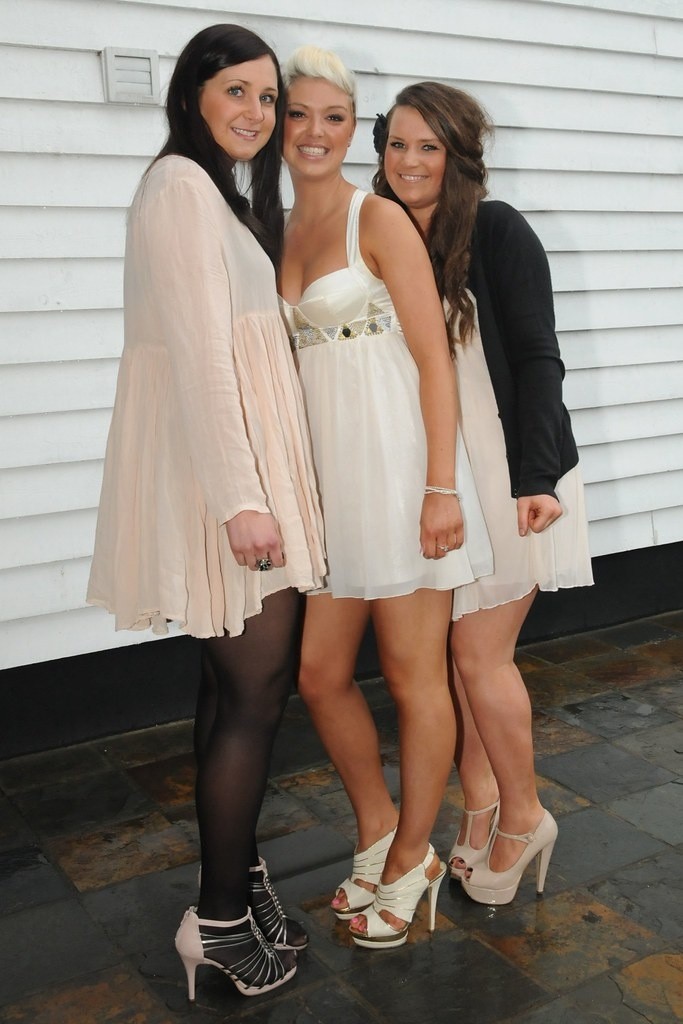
[424,485,458,496]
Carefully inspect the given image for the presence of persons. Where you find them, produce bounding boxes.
[85,22,330,1006]
[371,82,595,904]
[266,43,465,949]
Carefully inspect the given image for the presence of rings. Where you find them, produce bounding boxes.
[437,545,449,552]
[255,557,272,572]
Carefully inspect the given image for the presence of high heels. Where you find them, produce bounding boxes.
[175,906,297,1000]
[448,797,500,881]
[461,808,558,905]
[197,856,309,950]
[348,842,447,948]
[330,826,398,920]
